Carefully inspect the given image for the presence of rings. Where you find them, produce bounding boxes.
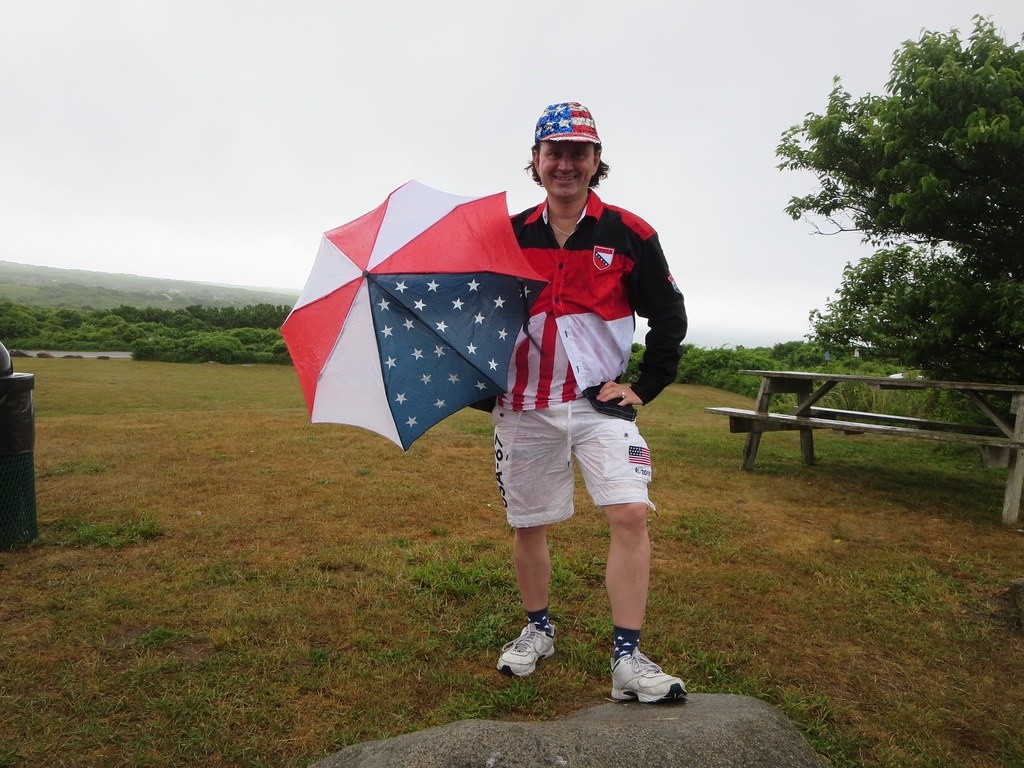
[622,390,625,398]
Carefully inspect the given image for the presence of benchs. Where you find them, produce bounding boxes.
[708,404,1024,524]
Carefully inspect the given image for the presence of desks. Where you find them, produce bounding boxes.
[736,369,1024,523]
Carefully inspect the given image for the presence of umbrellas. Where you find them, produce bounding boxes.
[281,178,550,451]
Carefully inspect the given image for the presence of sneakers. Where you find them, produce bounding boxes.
[497,623,555,677]
[610,647,687,704]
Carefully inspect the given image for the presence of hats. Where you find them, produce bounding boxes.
[535,102,603,144]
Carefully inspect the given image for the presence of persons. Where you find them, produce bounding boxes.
[493,102,687,706]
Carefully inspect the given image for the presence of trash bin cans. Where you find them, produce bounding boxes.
[0,340,39,552]
